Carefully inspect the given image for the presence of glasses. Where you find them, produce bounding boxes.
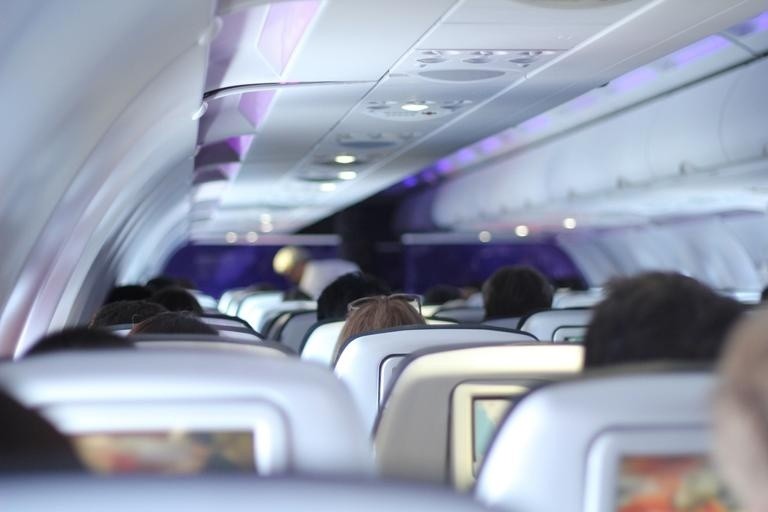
[347,293,421,314]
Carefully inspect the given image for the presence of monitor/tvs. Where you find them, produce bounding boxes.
[32,403,287,479]
[555,329,593,350]
[453,386,515,480]
[584,432,741,512]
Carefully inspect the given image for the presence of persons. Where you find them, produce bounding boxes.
[584,269,768,512]
[0,276,218,475]
[423,265,588,319]
[286,257,426,363]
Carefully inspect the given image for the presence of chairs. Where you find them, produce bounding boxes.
[0,288,768,511]
